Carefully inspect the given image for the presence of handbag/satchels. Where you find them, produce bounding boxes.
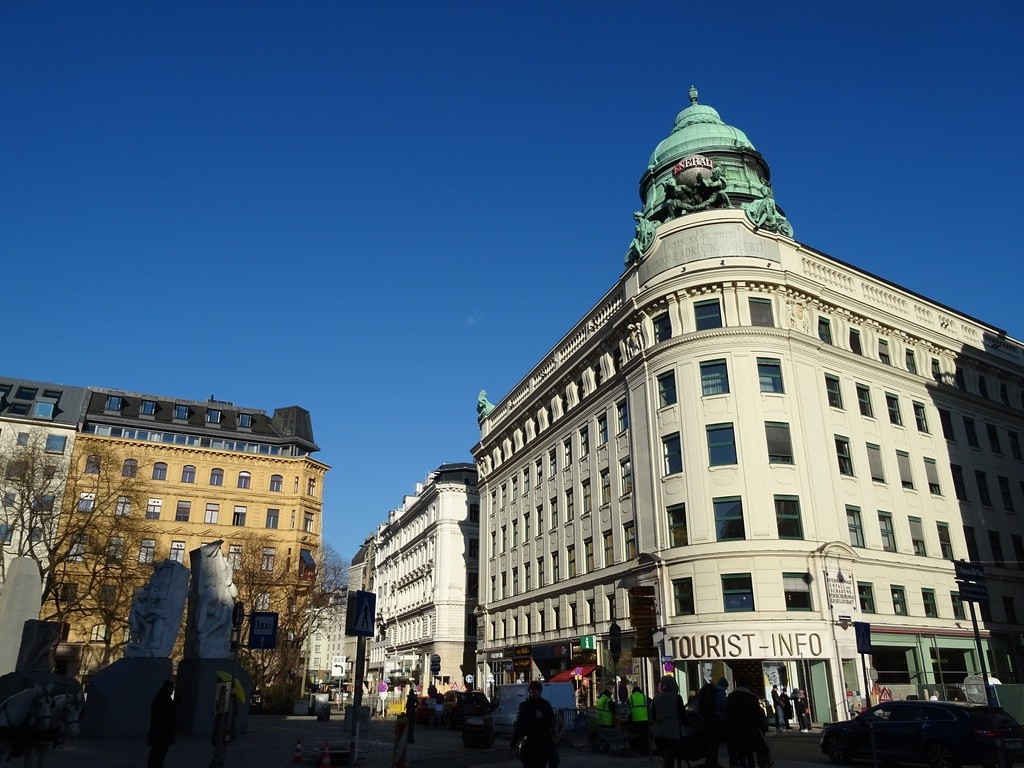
[548,723,560,767]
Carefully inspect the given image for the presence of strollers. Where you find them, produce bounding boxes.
[677,709,718,768]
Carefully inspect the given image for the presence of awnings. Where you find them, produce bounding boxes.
[550,665,599,682]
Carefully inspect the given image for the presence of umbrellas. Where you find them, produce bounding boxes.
[215,669,247,703]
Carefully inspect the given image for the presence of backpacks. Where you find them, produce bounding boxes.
[698,684,722,718]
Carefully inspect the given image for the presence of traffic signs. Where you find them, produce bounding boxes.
[332,655,345,676]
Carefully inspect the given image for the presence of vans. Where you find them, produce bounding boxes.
[489,682,576,737]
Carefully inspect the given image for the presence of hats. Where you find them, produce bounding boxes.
[719,678,729,687]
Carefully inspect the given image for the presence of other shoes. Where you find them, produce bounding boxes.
[801,729,809,732]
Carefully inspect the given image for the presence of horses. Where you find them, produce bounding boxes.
[0,681,85,768]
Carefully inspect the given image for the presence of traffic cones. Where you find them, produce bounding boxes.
[319,743,332,768]
[292,736,303,762]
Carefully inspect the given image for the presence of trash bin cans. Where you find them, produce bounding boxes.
[317,702,331,722]
[462,715,495,749]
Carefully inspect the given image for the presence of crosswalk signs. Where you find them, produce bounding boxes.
[354,590,375,637]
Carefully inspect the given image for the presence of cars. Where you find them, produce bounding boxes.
[440,690,491,729]
[819,700,1024,767]
[462,715,496,749]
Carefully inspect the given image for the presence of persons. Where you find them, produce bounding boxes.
[211,678,239,767]
[404,690,420,716]
[929,690,940,700]
[145,680,178,768]
[701,662,714,686]
[477,389,489,421]
[754,187,780,231]
[624,212,652,260]
[663,166,734,225]
[510,677,813,768]
